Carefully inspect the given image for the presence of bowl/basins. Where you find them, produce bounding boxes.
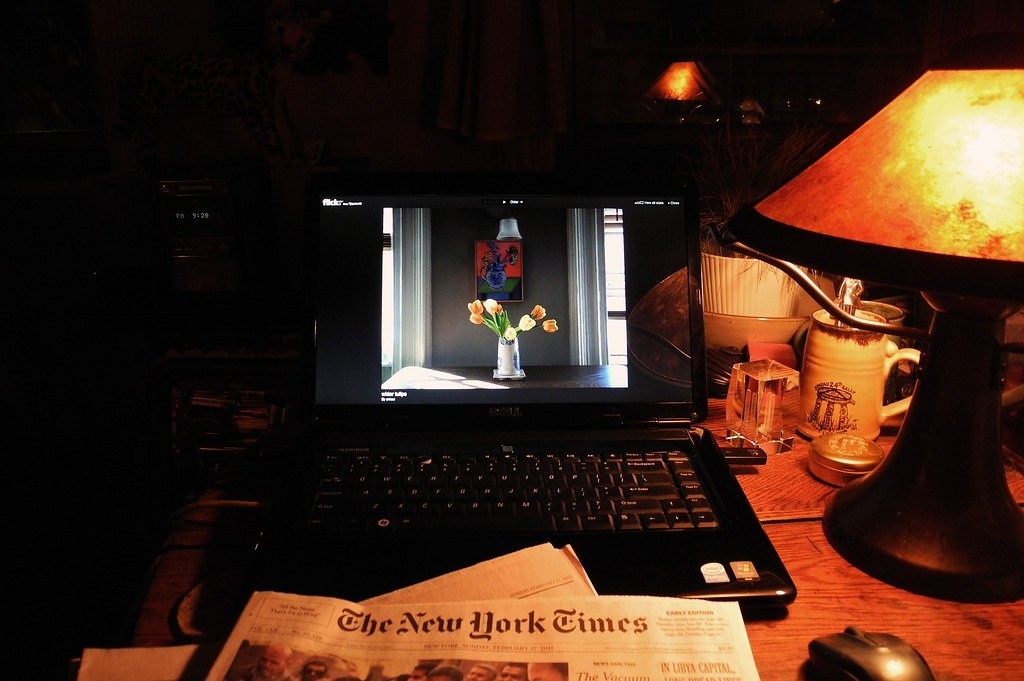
[704,312,811,355]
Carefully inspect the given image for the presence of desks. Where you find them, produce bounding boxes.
[129,380,1024,681]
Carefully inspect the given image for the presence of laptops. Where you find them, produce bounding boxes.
[250,168,799,616]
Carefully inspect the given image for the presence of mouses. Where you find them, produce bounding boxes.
[807,624,936,681]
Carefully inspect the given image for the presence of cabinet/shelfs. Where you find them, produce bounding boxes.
[1,0,153,318]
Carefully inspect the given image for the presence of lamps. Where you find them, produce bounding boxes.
[645,61,713,127]
[710,32,1024,603]
[496,207,522,241]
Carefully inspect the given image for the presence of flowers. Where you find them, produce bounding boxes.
[468,299,558,338]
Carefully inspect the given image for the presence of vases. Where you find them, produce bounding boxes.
[498,337,521,376]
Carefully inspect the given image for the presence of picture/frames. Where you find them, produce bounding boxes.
[475,240,524,302]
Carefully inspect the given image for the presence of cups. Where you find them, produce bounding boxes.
[796,307,921,442]
[859,299,905,356]
